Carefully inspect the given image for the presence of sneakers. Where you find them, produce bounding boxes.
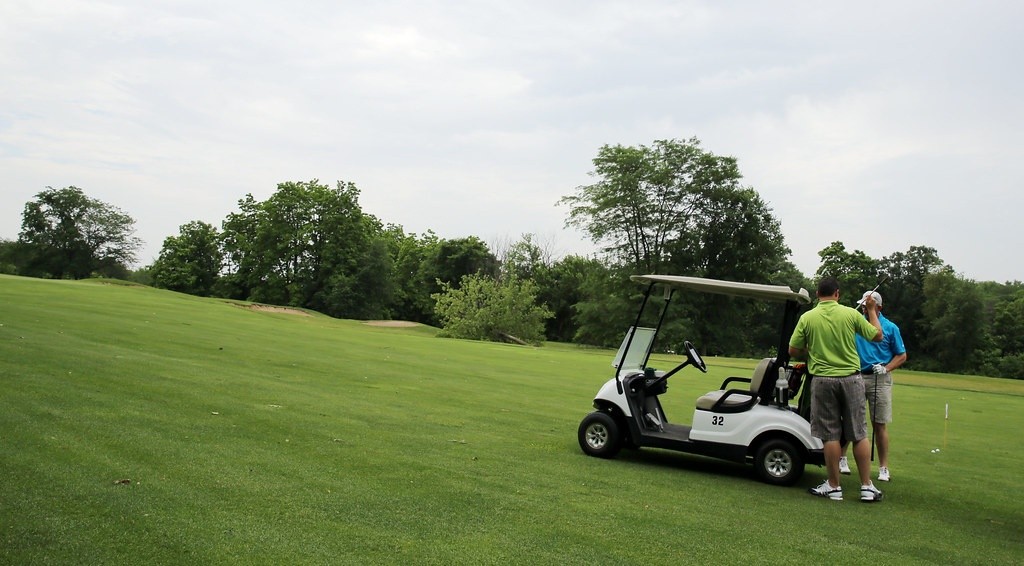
[807,479,843,501]
[860,479,882,501]
[839,456,851,473]
[877,466,890,481]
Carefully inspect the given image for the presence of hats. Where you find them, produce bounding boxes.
[857,291,882,307]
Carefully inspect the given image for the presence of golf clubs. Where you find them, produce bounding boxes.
[855,275,890,310]
[870,360,884,462]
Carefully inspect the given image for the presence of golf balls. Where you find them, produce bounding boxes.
[931,449,940,453]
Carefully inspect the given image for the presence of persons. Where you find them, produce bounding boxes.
[836,290,908,483]
[788,277,883,502]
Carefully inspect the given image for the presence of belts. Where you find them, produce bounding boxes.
[861,369,873,375]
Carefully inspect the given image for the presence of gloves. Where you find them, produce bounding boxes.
[871,363,887,375]
